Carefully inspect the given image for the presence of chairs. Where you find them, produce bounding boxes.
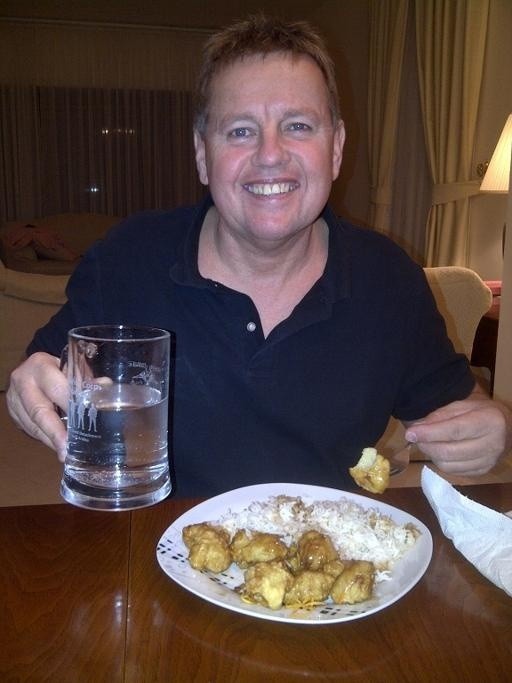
[379,266,495,477]
[1,260,67,509]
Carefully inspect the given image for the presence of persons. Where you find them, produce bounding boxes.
[2,8,509,500]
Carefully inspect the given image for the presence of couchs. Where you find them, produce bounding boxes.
[0,212,125,276]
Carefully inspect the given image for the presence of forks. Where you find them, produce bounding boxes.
[388,440,414,478]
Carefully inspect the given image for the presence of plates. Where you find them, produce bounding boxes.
[153,481,434,626]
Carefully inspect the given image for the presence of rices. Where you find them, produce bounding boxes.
[214,495,417,583]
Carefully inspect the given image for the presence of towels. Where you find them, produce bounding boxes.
[419,465,512,598]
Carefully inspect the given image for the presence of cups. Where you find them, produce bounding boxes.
[54,323,174,513]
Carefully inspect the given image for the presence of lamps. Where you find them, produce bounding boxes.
[479,113,512,192]
